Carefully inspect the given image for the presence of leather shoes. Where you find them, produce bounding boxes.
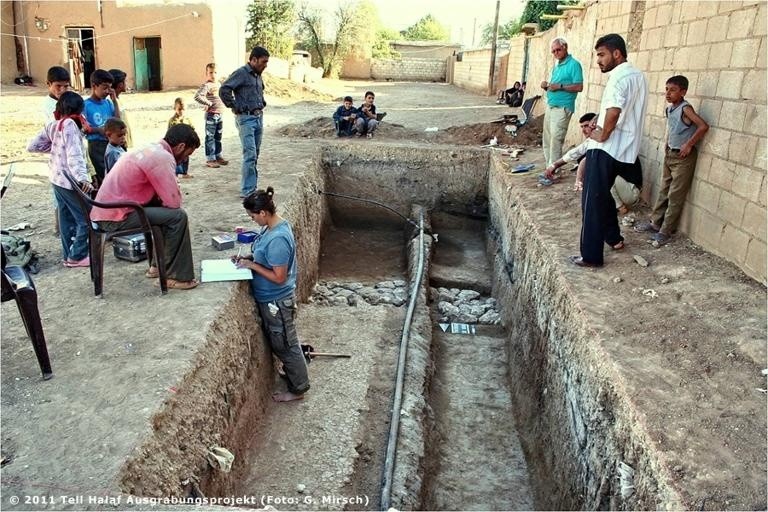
[569,255,602,267]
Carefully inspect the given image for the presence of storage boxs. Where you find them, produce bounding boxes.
[113,231,153,262]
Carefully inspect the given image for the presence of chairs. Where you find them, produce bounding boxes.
[64,168,170,296]
[1,160,19,201]
[0,233,60,380]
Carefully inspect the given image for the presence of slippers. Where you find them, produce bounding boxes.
[635,222,659,233]
[145,271,199,289]
[650,231,673,245]
[63,256,90,267]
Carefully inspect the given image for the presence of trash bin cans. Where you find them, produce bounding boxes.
[290,65,305,82]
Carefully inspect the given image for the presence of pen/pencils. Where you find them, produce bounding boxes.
[235,247,242,265]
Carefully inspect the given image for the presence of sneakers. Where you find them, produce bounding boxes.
[539,171,560,186]
[206,159,228,168]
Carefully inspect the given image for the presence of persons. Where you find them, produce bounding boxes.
[194,63,228,168]
[539,33,709,269]
[352,92,377,139]
[84,45,94,86]
[219,46,270,199]
[231,186,310,402]
[333,96,357,138]
[508,81,527,107]
[27,63,200,290]
[495,81,520,106]
